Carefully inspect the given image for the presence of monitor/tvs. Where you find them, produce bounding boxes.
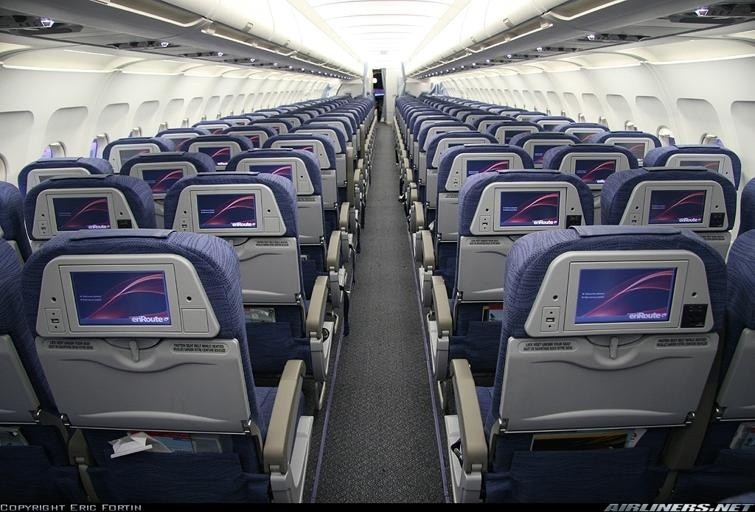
[641,185,712,228]
[190,190,263,233]
[35,97,373,199]
[564,260,688,330]
[494,187,567,231]
[58,264,181,332]
[397,95,725,187]
[46,193,117,235]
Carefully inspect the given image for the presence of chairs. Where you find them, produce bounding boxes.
[0,93,377,504]
[392,90,755,504]
